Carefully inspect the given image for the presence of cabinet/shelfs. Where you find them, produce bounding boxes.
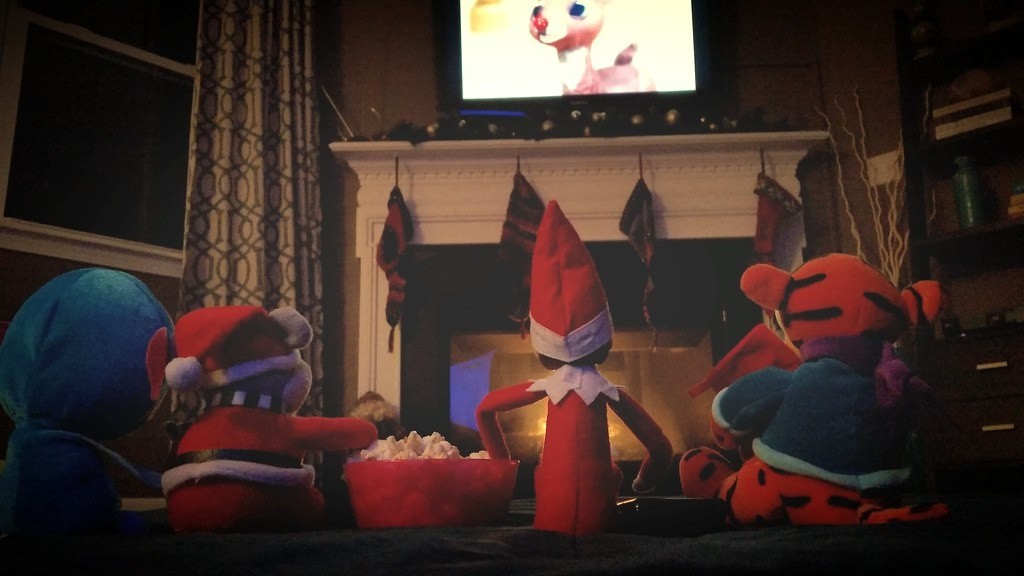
[892,0,1024,464]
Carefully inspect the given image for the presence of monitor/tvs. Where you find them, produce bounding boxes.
[431,0,726,123]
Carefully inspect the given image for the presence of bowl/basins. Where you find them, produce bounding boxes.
[345,457,520,529]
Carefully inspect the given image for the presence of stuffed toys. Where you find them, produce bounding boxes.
[476,200,672,530]
[0,268,175,537]
[160,303,379,535]
[679,253,948,525]
